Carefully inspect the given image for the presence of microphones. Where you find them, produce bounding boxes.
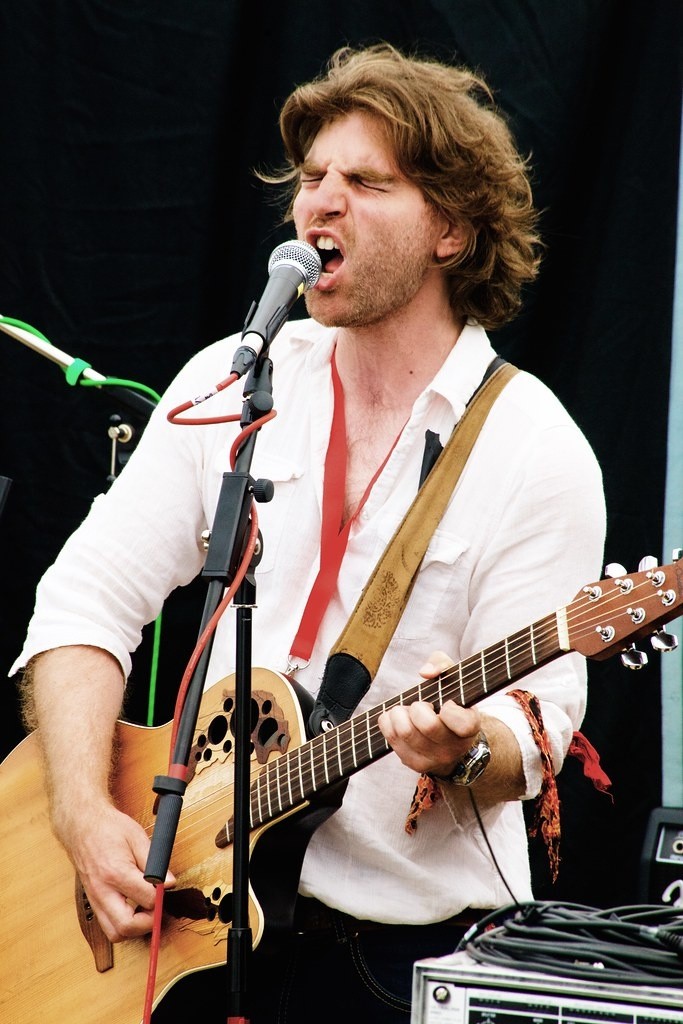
[230,239,321,380]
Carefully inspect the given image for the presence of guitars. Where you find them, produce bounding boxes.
[0,543,683,1023]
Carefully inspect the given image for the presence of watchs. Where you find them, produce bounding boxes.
[444,719,494,791]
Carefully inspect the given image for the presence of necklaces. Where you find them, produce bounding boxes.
[277,329,416,683]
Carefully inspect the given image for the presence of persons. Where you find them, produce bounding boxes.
[8,41,610,1024]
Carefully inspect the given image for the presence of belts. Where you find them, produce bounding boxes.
[303,899,476,931]
